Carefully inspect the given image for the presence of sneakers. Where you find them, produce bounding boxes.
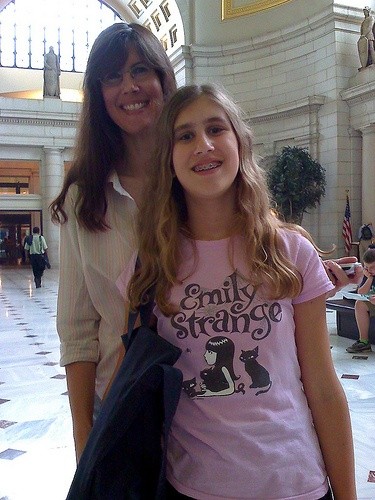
[346,339,372,353]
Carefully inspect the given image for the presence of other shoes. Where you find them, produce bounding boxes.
[36,284,42,288]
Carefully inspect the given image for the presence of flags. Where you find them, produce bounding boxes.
[342,189,353,256]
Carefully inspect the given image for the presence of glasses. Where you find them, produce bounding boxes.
[97,62,155,87]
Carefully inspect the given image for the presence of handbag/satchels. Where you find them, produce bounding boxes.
[65,247,184,500]
[40,254,48,265]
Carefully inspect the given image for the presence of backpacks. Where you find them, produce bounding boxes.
[358,224,372,241]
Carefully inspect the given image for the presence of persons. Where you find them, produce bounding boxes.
[360,5,375,65]
[48,21,364,467]
[345,222,375,353]
[0,227,51,288]
[102,83,358,500]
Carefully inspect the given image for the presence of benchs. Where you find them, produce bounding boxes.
[326,299,375,345]
